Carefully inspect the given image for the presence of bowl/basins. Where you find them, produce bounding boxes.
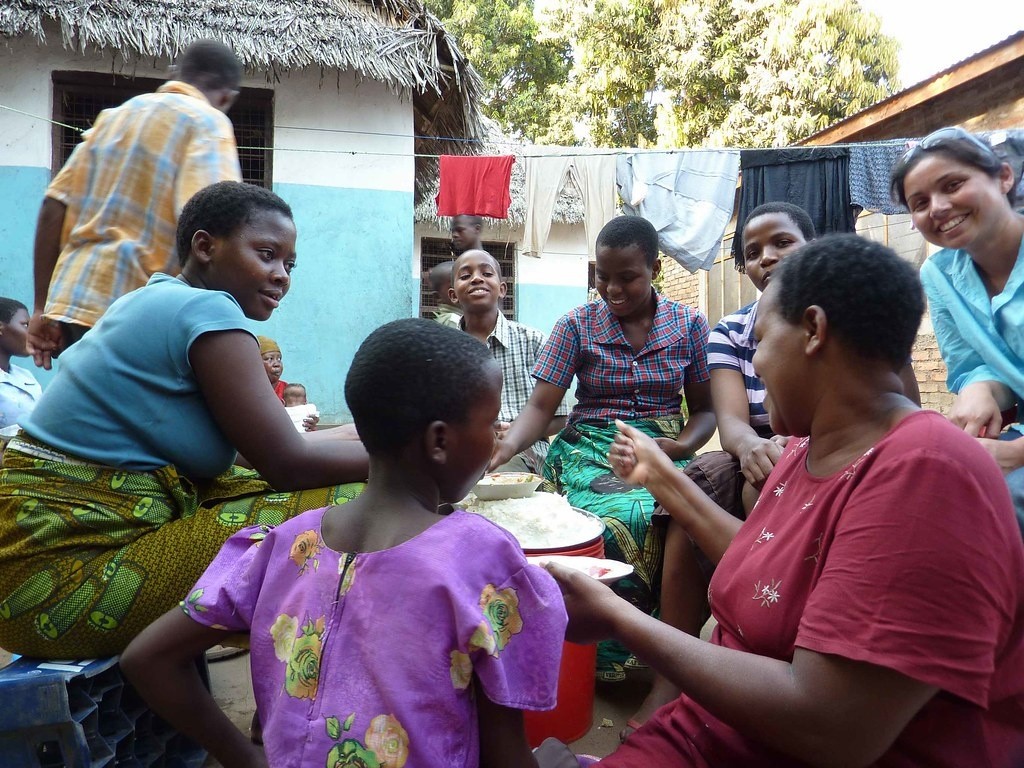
[472,472,545,501]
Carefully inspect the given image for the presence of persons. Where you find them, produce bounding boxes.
[619,203,921,741]
[0,297,42,463]
[891,138,1024,540]
[437,250,568,475]
[281,384,317,433]
[485,215,718,681]
[420,213,483,284]
[257,336,319,431]
[25,41,243,370]
[430,261,464,329]
[539,235,1024,768]
[119,317,597,768]
[0,181,510,744]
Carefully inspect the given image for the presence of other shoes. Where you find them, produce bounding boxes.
[205,644,246,662]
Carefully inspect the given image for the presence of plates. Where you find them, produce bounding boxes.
[527,554,635,586]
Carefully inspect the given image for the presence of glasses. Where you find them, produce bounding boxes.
[898,127,995,163]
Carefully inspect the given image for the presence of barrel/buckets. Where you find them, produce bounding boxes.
[524,537,609,753]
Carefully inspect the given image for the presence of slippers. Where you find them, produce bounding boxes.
[619,712,650,742]
